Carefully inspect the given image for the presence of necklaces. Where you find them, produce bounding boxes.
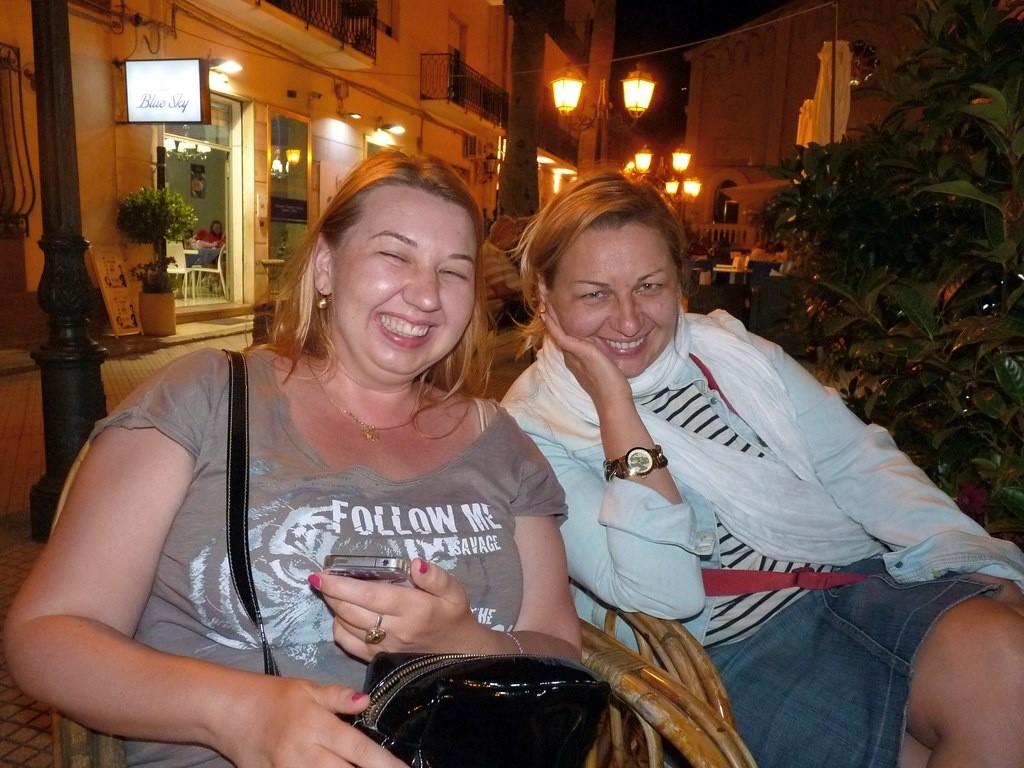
[304,351,423,444]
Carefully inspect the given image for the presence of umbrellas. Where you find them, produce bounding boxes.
[794,36,854,157]
[719,178,802,207]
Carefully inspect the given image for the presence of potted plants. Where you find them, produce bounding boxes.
[114,187,198,337]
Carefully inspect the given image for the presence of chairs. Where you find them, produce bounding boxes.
[50,427,757,768]
[165,241,192,304]
[192,243,226,300]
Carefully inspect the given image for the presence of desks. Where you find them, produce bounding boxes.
[184,250,227,255]
[712,267,753,289]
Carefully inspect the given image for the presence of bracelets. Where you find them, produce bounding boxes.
[502,631,524,656]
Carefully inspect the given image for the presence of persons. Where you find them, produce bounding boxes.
[495,165,1024,768]
[187,220,226,252]
[480,212,531,329]
[0,150,583,768]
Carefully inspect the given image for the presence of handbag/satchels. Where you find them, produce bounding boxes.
[344,653,610,768]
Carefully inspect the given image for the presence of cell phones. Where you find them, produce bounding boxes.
[323,554,416,589]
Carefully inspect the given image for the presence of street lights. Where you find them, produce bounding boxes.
[551,59,660,167]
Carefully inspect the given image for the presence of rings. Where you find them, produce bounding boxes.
[363,612,387,647]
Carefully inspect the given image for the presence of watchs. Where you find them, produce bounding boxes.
[603,444,669,481]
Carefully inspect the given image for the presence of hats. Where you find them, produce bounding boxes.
[196,229,209,240]
